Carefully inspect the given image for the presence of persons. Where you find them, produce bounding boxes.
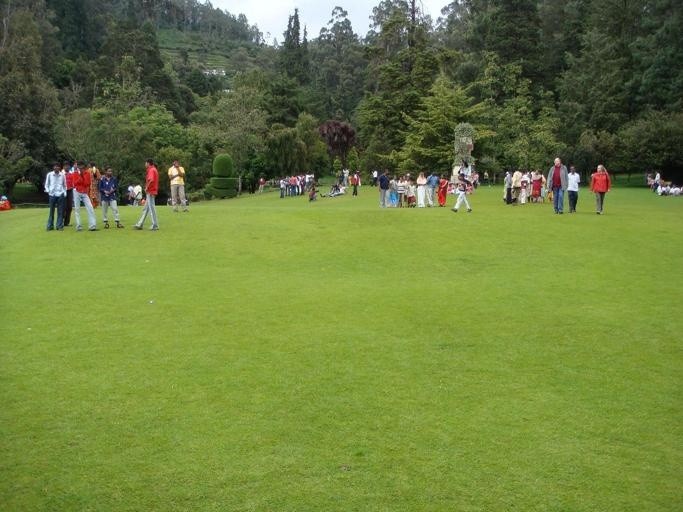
[44,157,188,232]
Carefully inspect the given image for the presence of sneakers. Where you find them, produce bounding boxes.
[467,208,471,212]
[597,211,601,214]
[451,208,457,212]
[47,224,142,231]
[554,210,576,214]
